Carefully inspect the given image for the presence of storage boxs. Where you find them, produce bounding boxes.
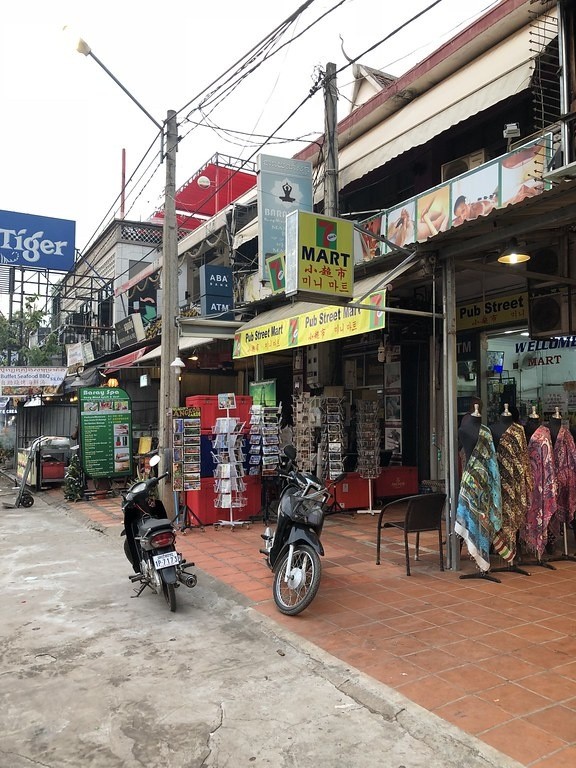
[42,461,65,479]
[185,393,253,430]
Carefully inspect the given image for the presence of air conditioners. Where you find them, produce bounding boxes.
[61,332,86,347]
[90,334,111,350]
[521,237,576,289]
[440,148,494,184]
[526,289,576,338]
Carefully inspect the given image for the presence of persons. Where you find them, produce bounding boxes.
[454,402,576,572]
[362,190,497,260]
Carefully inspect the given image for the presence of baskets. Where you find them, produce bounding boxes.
[282,495,328,528]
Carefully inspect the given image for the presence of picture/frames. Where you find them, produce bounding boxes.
[383,361,401,388]
[385,427,404,454]
[384,394,402,422]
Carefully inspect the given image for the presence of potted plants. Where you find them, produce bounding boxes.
[95,478,111,499]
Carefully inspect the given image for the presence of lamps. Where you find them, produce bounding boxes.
[497,236,531,264]
[377,330,385,362]
[169,325,186,374]
[503,122,520,146]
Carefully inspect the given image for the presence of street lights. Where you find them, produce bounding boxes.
[63,25,177,525]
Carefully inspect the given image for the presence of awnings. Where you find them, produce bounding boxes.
[133,338,217,364]
[233,260,420,360]
[103,344,160,373]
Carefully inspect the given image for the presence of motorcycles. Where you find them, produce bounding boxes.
[259,445,347,617]
[112,454,198,611]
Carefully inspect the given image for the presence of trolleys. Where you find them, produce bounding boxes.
[2,435,48,508]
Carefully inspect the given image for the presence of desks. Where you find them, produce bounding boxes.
[186,467,418,527]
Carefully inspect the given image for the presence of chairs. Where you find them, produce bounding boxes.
[376,493,447,576]
